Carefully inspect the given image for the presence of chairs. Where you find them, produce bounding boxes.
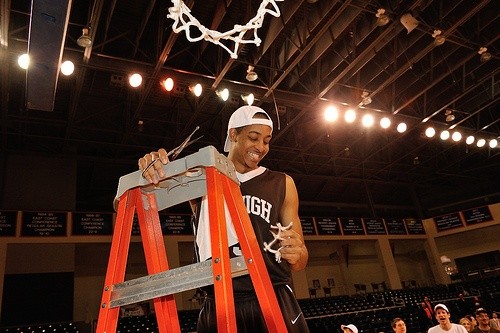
[0,276,500,333]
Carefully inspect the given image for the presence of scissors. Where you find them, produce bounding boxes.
[139,124,204,179]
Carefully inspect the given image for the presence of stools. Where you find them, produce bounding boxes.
[96,145,288,333]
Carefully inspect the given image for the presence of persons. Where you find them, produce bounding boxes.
[341,324,358,333]
[137,105,310,333]
[391,317,407,333]
[428,303,469,333]
[459,307,500,333]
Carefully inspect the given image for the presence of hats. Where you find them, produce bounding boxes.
[434,303,449,312]
[223,105,274,152]
[474,308,487,314]
[340,324,358,333]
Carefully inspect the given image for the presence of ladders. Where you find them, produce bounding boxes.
[94,145,291,333]
